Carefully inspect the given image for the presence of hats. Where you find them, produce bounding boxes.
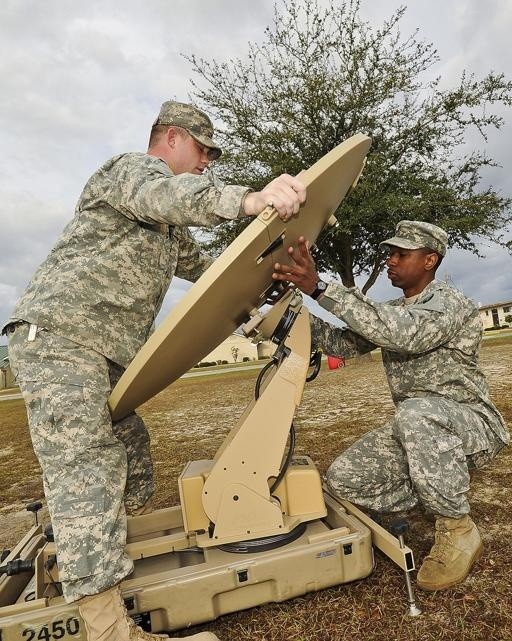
[153,101,222,160]
[379,220,450,257]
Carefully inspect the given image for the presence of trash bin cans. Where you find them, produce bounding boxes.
[328,355,345,369]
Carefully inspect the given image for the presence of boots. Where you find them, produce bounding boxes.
[75,584,220,641]
[417,513,485,591]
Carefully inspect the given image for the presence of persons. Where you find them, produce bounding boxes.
[1,100,308,641]
[271,219,511,592]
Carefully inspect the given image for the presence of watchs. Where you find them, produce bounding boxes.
[310,280,329,300]
